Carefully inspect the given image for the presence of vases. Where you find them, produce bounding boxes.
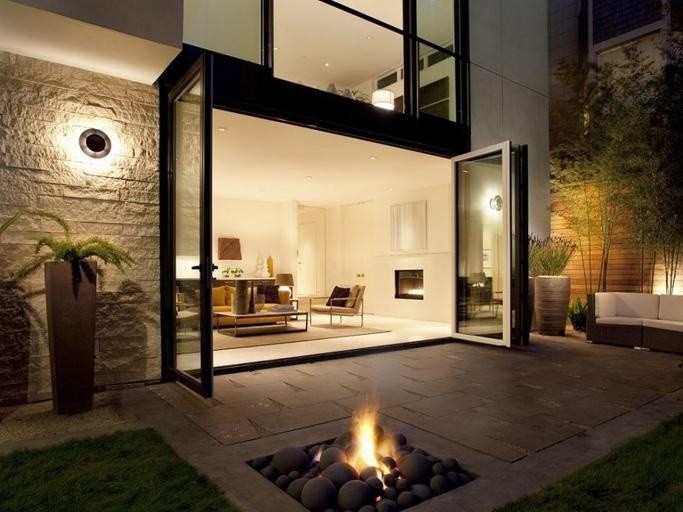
[528,275,572,336]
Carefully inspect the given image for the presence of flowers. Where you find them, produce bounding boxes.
[528,231,578,277]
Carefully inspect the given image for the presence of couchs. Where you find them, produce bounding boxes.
[587,292,683,355]
[185,285,291,328]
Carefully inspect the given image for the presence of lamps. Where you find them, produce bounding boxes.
[273,273,293,305]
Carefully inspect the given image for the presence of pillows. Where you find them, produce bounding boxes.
[325,285,358,307]
[210,285,256,305]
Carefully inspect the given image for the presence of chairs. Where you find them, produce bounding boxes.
[310,284,366,329]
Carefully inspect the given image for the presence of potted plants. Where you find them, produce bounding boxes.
[0,205,138,417]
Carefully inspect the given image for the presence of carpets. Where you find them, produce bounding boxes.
[175,318,391,355]
